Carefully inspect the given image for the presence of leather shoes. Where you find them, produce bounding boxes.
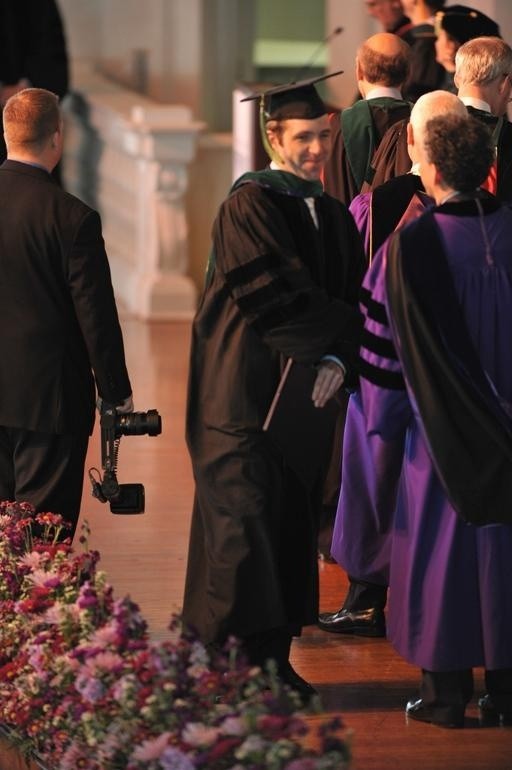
[478,693,511,722]
[405,697,463,728]
[263,657,317,708]
[318,607,384,637]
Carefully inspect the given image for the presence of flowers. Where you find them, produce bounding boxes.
[1,498,354,770]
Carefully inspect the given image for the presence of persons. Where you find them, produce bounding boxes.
[309,0,512,727]
[0,86,135,587]
[0,0,74,194]
[181,69,369,711]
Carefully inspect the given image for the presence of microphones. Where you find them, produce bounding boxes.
[292,26,344,84]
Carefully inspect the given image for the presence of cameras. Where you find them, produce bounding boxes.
[87,399,162,515]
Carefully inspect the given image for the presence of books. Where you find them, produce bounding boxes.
[263,358,340,491]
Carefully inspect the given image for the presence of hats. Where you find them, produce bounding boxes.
[240,71,342,165]
